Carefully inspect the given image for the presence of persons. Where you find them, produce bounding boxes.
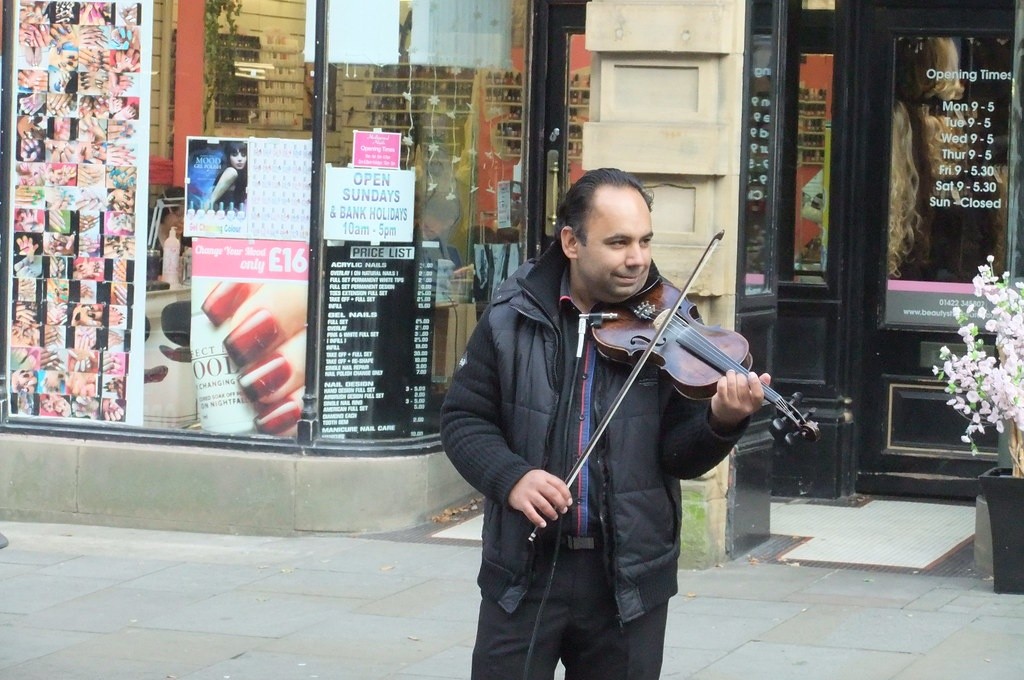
[803,169,823,267]
[438,168,771,680]
[147,186,192,282]
[205,142,247,212]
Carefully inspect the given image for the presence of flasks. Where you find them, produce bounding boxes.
[147,250,160,281]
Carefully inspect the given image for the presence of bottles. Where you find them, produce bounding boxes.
[162,227,180,289]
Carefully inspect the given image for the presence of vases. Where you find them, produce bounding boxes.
[978,466,1024,595]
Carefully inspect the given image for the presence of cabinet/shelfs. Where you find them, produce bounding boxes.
[481,70,592,159]
[211,34,262,131]
[260,32,307,130]
[795,99,825,167]
[325,63,478,204]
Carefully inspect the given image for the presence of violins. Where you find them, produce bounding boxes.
[589,277,826,439]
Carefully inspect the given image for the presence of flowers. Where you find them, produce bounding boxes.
[931,254,1024,477]
